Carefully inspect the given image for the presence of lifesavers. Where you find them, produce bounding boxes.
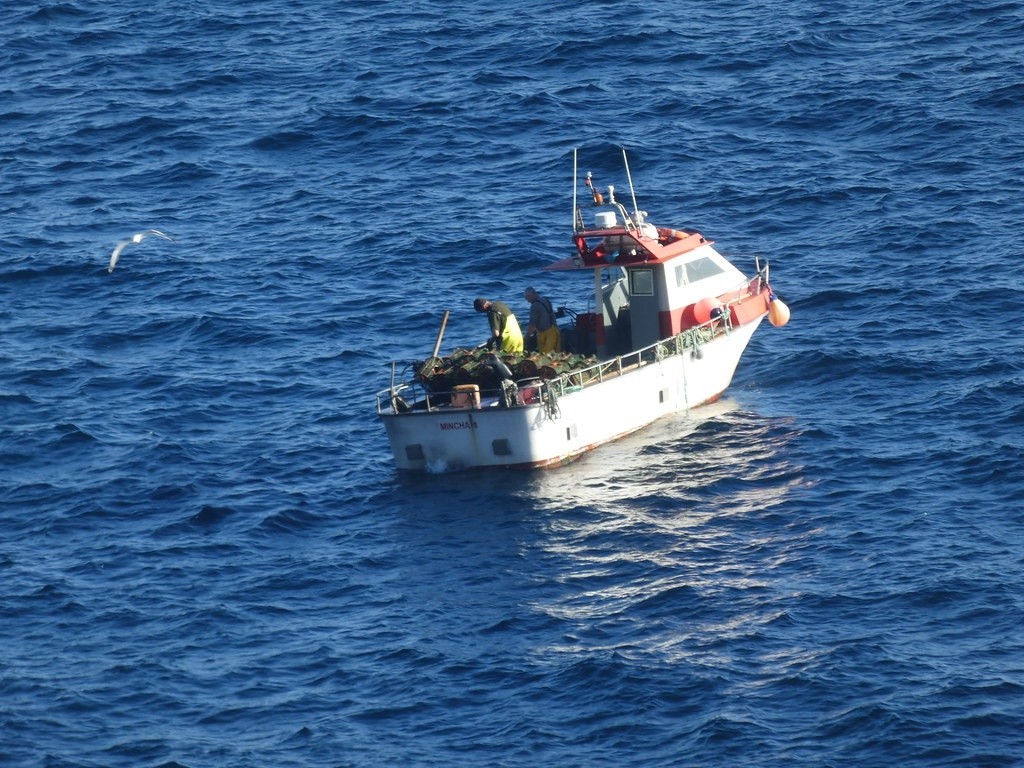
[658,227,690,241]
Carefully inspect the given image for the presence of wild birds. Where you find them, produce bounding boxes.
[108,229,174,275]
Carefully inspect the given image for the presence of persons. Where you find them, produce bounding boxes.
[523,287,561,353]
[473,298,523,352]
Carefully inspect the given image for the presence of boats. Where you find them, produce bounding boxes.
[378,141,772,476]
